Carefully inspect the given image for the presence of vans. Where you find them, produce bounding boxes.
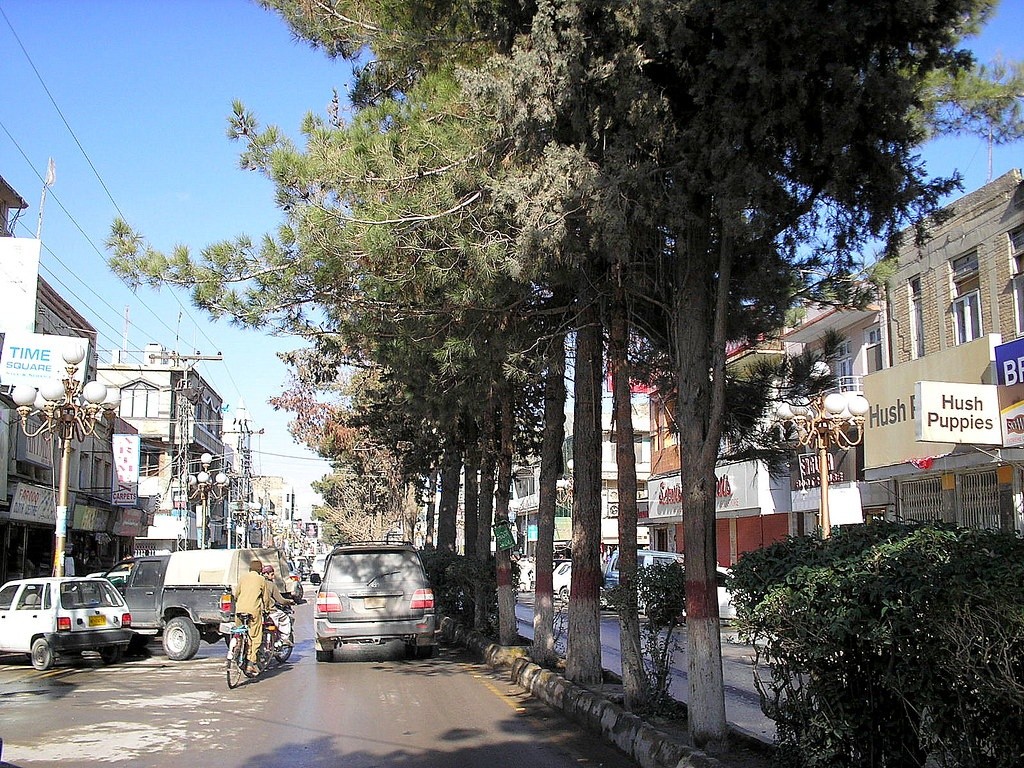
[517,558,571,588]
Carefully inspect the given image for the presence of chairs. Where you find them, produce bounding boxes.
[61,593,74,608]
[22,593,42,609]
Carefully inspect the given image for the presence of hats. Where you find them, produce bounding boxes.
[262,565,274,574]
[249,561,262,573]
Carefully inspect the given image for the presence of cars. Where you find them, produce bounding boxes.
[602,549,686,603]
[715,568,764,620]
[0,578,132,671]
[71,571,130,598]
[552,562,573,604]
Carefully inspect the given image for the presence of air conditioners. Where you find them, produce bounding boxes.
[606,489,619,518]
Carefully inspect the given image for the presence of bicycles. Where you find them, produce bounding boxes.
[216,611,274,690]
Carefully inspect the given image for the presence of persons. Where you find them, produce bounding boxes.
[260,565,296,646]
[234,561,271,673]
[83,548,102,575]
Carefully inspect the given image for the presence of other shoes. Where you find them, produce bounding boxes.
[247,663,261,673]
[283,640,295,646]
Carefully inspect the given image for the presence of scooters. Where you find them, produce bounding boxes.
[229,601,299,665]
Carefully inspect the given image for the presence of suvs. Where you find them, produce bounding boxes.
[309,540,437,661]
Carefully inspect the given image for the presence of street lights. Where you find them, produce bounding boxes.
[185,452,230,550]
[9,342,121,580]
[777,361,870,542]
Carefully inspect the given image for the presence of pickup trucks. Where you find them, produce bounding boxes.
[62,549,288,661]
